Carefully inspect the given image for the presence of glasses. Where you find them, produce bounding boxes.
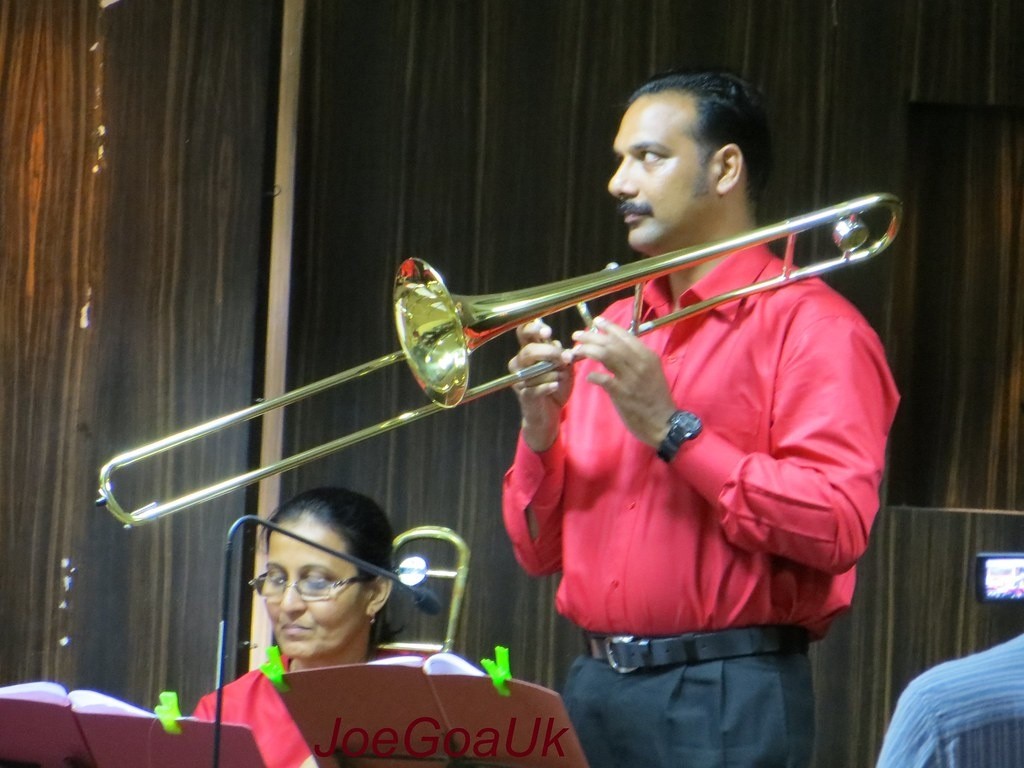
[248,573,375,600]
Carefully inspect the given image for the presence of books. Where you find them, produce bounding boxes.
[279,652,590,767]
[0,681,267,767]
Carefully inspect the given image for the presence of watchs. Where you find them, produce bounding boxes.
[656,410,702,464]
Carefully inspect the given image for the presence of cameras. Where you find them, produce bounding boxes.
[974,553,1024,604]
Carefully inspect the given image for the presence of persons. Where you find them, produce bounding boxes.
[500,74,898,766]
[191,487,427,765]
[878,632,1023,767]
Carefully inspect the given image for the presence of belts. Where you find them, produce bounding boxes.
[583,624,811,674]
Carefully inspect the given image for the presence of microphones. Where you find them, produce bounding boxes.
[215,515,441,768]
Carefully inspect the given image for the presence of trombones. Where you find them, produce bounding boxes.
[94,192,904,534]
[377,522,476,659]
